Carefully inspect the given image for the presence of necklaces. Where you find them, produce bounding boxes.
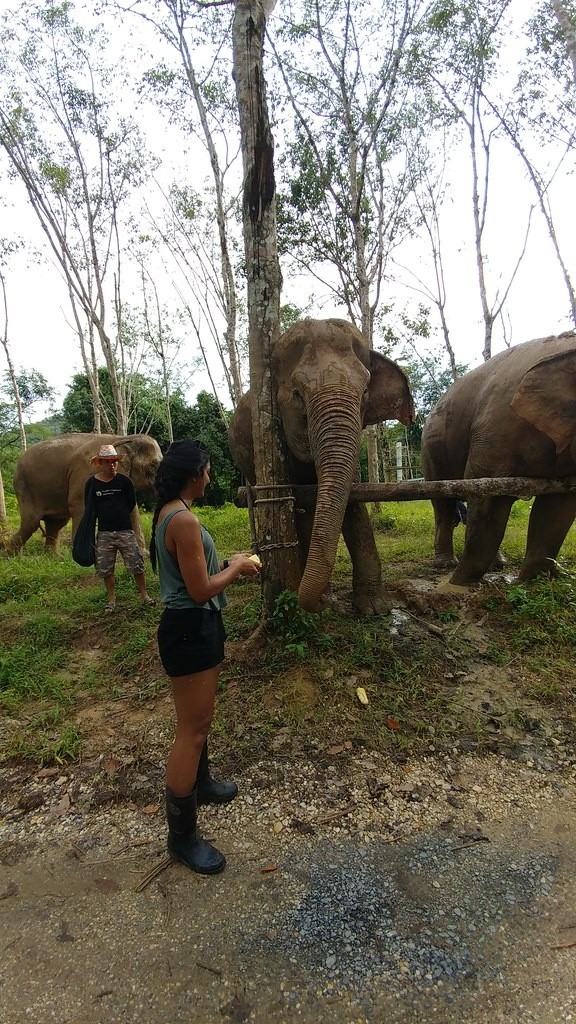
[177,493,209,531]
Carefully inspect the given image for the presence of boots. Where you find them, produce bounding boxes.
[166,783,225,873]
[197,739,238,805]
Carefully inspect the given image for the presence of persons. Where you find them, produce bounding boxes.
[150,440,263,876]
[84,444,155,612]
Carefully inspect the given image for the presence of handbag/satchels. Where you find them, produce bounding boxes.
[72,476,95,567]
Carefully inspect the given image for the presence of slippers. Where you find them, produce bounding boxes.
[104,604,115,612]
[144,599,156,606]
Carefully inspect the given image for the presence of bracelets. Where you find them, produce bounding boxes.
[223,558,228,569]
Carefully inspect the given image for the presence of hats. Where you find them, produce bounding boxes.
[90,445,126,467]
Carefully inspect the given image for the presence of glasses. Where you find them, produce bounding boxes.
[194,440,206,454]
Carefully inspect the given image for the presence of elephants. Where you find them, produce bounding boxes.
[227,318,576,616]
[0,433,164,557]
